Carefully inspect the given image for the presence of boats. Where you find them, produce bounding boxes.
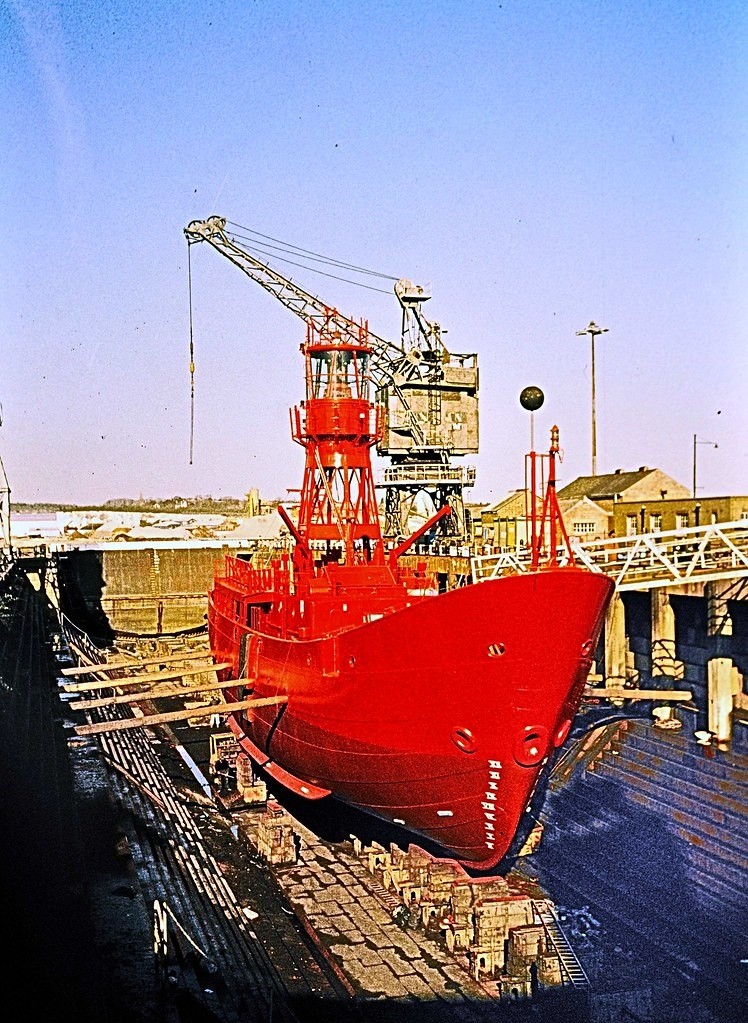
[208,305,620,873]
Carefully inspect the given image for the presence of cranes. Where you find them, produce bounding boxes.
[183,216,478,591]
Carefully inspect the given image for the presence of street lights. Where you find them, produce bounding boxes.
[693,434,718,497]
[574,318,611,476]
